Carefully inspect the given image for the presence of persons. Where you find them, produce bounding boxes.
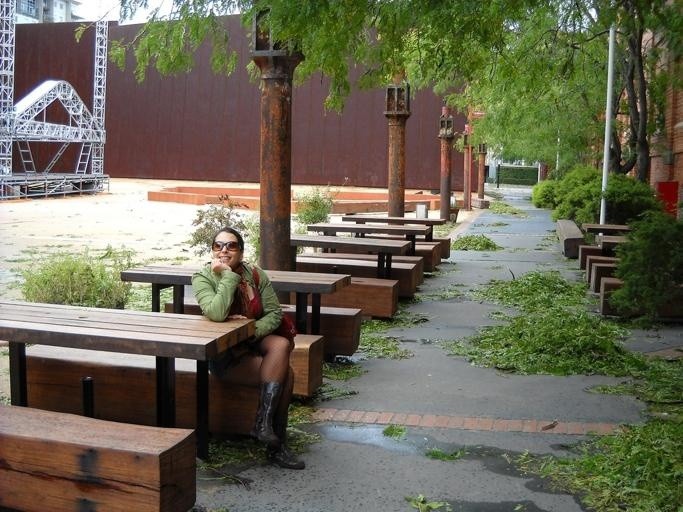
[191,227,305,468]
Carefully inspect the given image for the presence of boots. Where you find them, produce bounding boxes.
[267,414,307,471]
[249,380,282,445]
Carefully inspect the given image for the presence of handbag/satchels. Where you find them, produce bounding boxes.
[247,289,296,337]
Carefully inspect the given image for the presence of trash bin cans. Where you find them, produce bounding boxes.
[416,204,428,220]
[449,209,459,222]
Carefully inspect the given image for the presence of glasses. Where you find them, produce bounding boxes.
[210,241,242,252]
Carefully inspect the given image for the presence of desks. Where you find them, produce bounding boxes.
[123,265,352,336]
[342,213,446,242]
[290,235,411,279]
[1,301,256,466]
[307,222,431,256]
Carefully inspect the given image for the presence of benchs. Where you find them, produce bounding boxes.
[296,234,450,297]
[288,335,325,403]
[163,297,362,360]
[0,403,198,511]
[26,344,266,436]
[554,219,683,322]
[290,277,399,322]
